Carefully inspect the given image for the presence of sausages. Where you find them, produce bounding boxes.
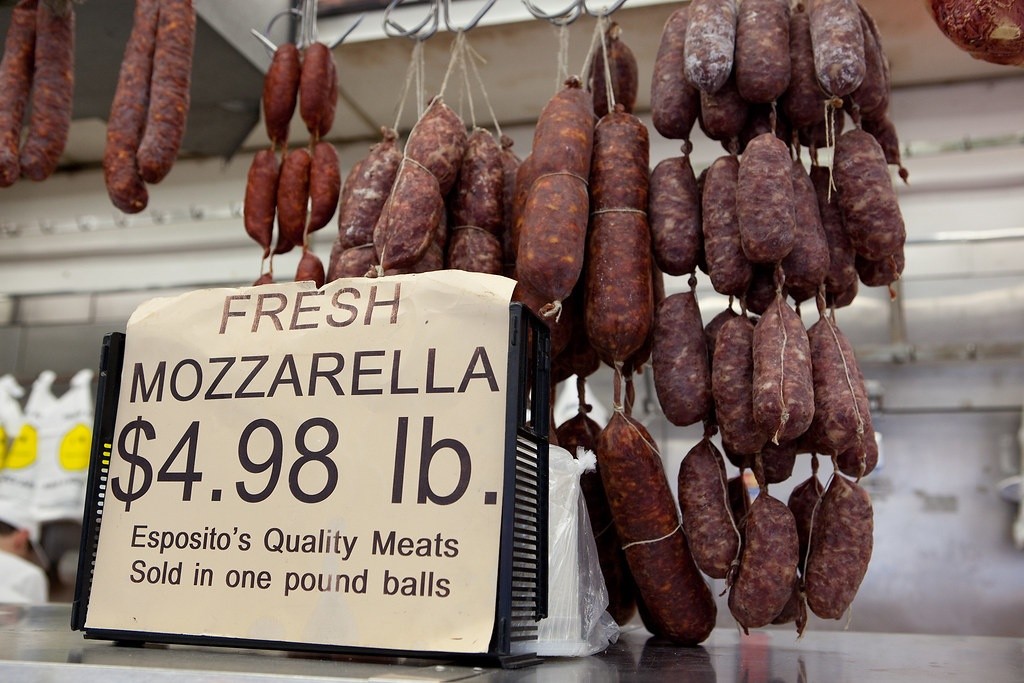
[103,0,194,214]
[243,0,908,650]
[0,1,76,188]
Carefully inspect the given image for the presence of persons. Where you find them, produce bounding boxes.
[0,506,51,606]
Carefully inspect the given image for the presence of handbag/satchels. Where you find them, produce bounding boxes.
[0,368,94,521]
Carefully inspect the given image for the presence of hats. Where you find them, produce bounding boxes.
[0,499,48,568]
